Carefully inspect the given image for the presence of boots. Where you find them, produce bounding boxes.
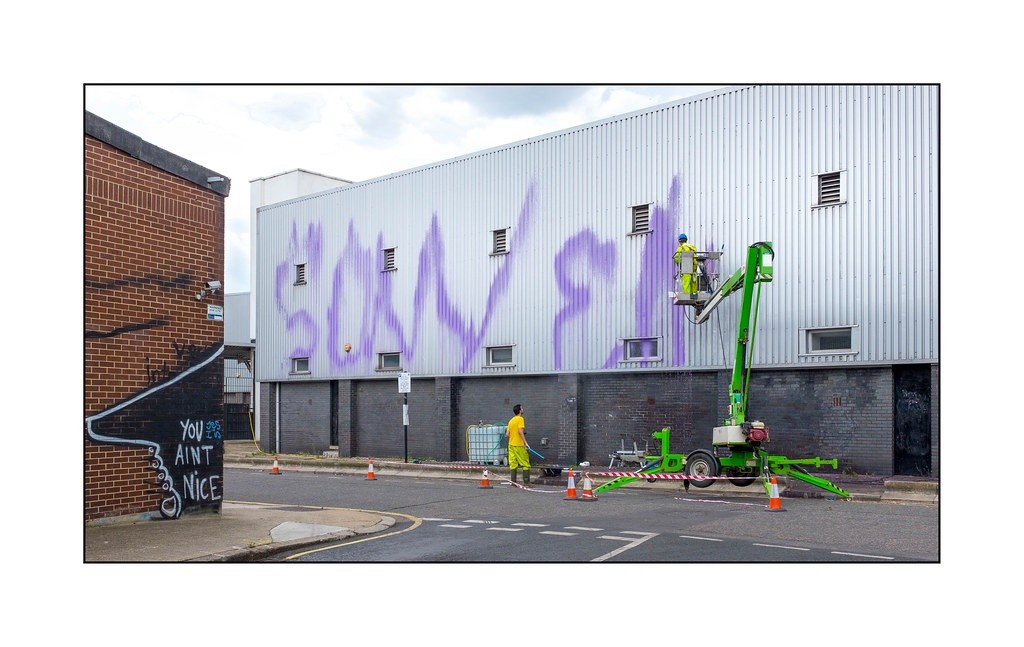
[523,470,536,488]
[510,469,519,485]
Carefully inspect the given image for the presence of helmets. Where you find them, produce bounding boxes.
[678,234,687,240]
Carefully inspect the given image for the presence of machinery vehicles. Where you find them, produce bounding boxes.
[642,239,853,500]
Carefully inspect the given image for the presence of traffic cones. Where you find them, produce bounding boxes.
[479,468,495,489]
[562,471,578,499]
[765,475,786,512]
[578,471,599,501]
[270,457,283,474]
[365,457,377,481]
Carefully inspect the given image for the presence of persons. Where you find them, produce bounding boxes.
[506,403,536,489]
[674,233,698,294]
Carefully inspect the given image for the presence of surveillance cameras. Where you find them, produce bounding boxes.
[202,281,221,291]
